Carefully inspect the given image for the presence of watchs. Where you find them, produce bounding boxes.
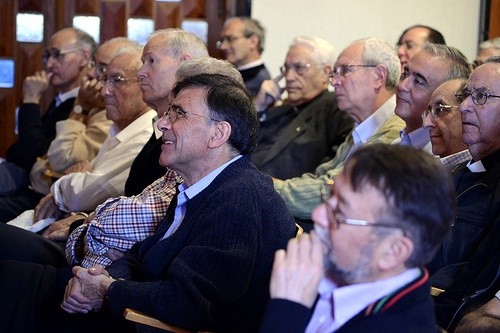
[73,105,88,115]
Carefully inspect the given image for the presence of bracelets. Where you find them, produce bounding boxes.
[76,212,88,219]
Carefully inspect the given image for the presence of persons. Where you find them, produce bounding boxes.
[1,15,500,332]
[261,144,457,333]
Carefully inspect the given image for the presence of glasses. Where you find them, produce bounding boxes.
[321,183,406,238]
[100,76,138,87]
[42,46,85,63]
[280,62,326,75]
[455,89,500,105]
[87,59,108,76]
[420,103,459,124]
[215,31,254,48]
[162,108,220,122]
[329,64,376,79]
